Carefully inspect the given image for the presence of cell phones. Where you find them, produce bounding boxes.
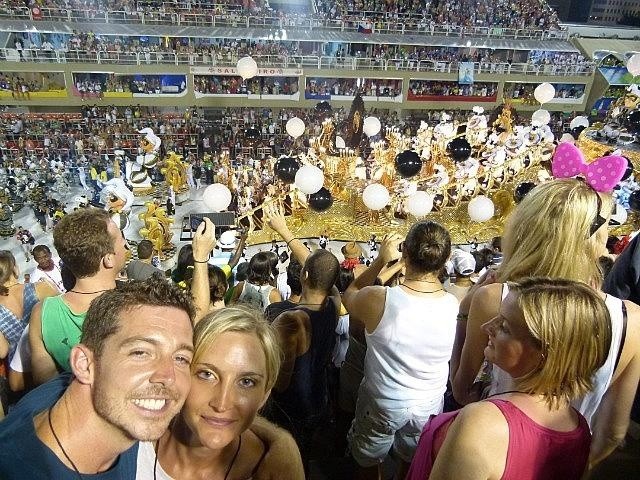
[399,242,404,252]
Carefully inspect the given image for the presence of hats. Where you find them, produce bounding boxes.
[341,241,363,258]
[216,231,236,249]
[451,249,476,275]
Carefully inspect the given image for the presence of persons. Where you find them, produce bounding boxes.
[0,1,640,479]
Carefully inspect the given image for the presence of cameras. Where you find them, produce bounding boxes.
[190,216,206,232]
